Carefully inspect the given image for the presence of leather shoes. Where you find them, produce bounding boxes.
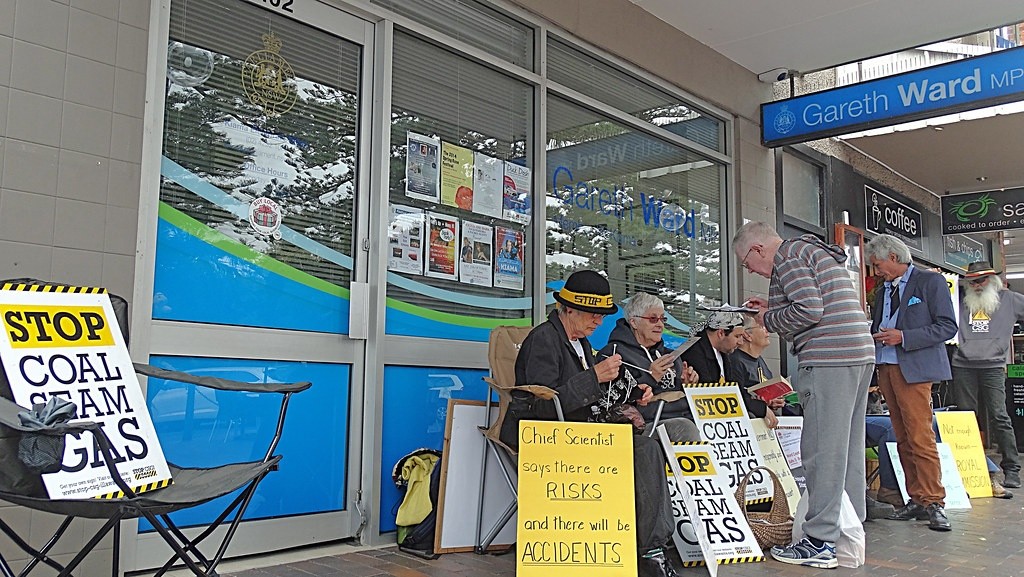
[925,503,951,531]
[886,500,929,520]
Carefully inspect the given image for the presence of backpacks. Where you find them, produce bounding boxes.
[389,447,441,560]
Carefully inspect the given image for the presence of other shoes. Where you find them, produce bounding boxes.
[878,487,904,505]
[866,495,895,518]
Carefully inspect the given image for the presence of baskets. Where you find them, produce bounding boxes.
[734,466,794,549]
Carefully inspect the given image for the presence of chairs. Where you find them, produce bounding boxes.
[474,325,567,555]
[0,277,313,577]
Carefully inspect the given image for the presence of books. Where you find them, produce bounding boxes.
[695,302,760,312]
[747,375,792,402]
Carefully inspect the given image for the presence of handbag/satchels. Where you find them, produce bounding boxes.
[792,484,863,568]
[587,402,646,437]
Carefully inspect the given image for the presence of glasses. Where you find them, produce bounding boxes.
[741,244,763,269]
[594,313,606,319]
[629,316,667,324]
[969,276,989,286]
[745,323,764,330]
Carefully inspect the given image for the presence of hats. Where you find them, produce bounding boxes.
[689,303,744,335]
[553,270,618,314]
[964,262,1002,281]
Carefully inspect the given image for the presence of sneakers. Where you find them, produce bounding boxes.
[989,473,1013,498]
[638,547,679,577]
[769,538,838,569]
[1003,473,1022,487]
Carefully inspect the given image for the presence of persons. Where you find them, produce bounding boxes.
[864,234,959,531]
[731,308,894,521]
[462,236,473,262]
[931,261,1024,488]
[683,309,780,429]
[595,292,700,443]
[476,243,490,264]
[731,221,875,569]
[867,370,970,508]
[503,239,515,259]
[500,270,682,577]
[986,455,1014,500]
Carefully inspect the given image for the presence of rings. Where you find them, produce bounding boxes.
[882,340,885,344]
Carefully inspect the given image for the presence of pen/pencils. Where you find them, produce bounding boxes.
[741,294,760,306]
